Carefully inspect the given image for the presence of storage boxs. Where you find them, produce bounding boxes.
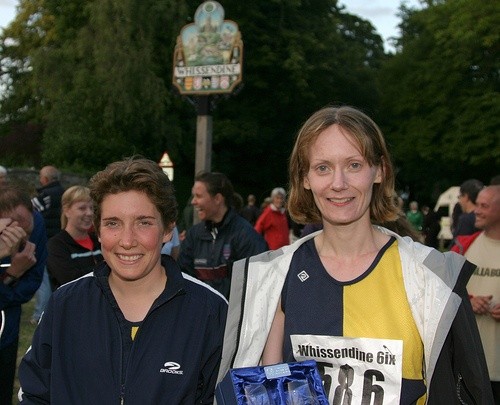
[215,359,330,405]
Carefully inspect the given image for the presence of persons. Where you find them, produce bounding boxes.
[241,188,441,251]
[0,166,46,405]
[27,165,67,325]
[449,184,500,405]
[15,159,228,405]
[176,171,269,301]
[47,185,105,291]
[214,107,495,405]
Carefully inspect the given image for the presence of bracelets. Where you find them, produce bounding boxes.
[5,271,18,281]
